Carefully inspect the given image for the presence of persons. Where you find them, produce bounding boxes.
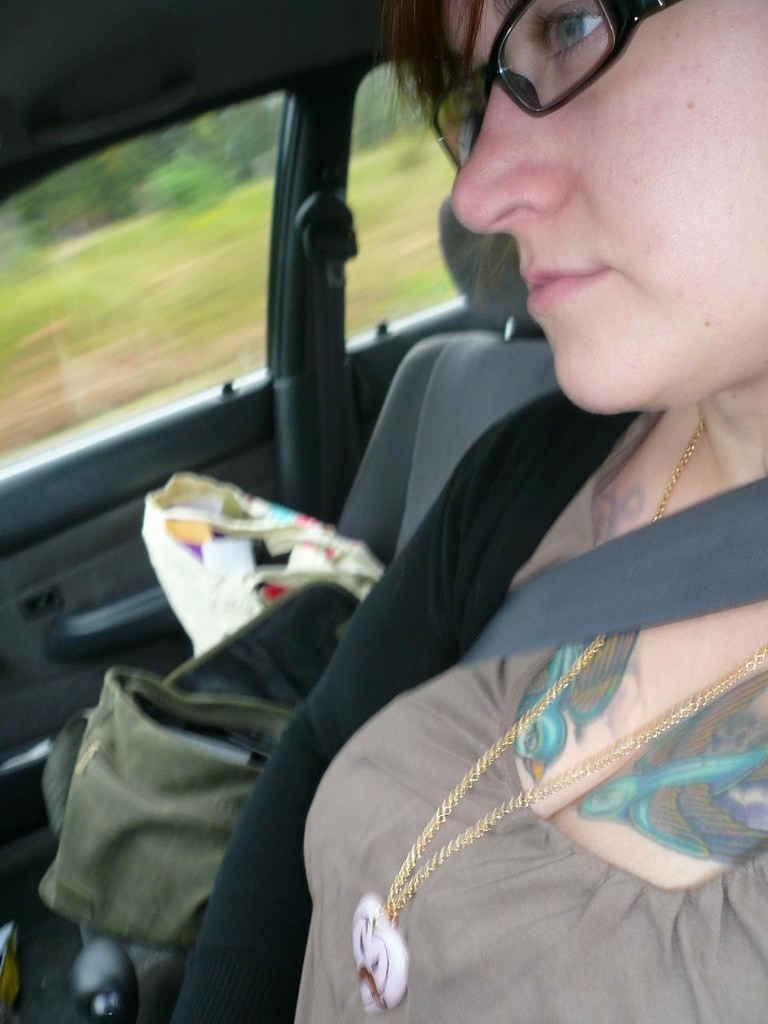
[292,1,768,1024]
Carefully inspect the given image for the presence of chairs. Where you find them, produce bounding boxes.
[0,197,558,1023]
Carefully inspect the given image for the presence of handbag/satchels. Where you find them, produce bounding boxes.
[38,580,369,945]
[143,468,385,660]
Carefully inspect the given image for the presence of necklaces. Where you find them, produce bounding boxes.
[353,423,768,1014]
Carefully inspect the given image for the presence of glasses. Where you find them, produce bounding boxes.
[430,0,682,173]
